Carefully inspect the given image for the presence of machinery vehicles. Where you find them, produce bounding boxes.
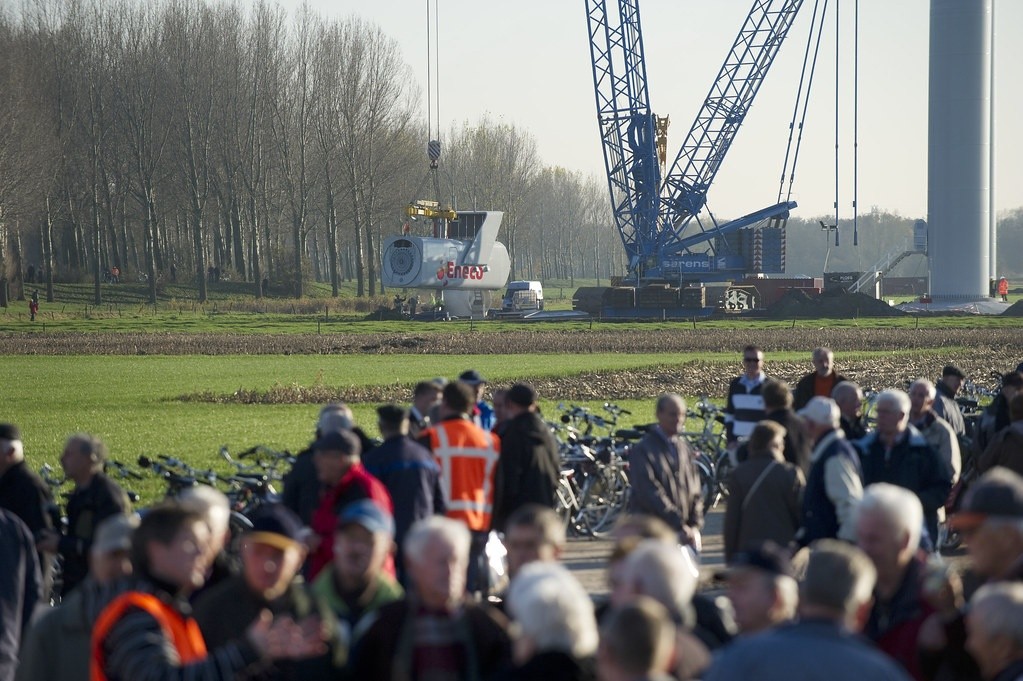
[407,0,858,320]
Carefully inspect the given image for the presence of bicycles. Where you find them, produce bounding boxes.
[545,399,728,540]
[38,444,296,610]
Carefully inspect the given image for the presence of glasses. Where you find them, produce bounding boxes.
[743,356,760,364]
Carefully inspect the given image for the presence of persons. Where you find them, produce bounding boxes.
[630,393,703,547]
[795,396,863,548]
[725,345,773,450]
[310,499,601,680]
[832,381,874,440]
[850,386,951,548]
[30,290,38,320]
[104,265,119,284]
[704,482,933,681]
[792,347,848,412]
[923,467,1023,681]
[394,292,436,319]
[748,381,807,473]
[278,370,561,595]
[170,263,268,297]
[724,418,806,564]
[933,364,972,472]
[977,361,1023,476]
[0,422,338,680]
[908,377,961,543]
[990,276,1008,301]
[596,515,738,681]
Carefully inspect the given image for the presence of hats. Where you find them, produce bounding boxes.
[92,518,139,557]
[311,431,359,457]
[336,498,395,540]
[246,504,308,553]
[943,365,967,380]
[458,369,488,386]
[797,396,840,427]
